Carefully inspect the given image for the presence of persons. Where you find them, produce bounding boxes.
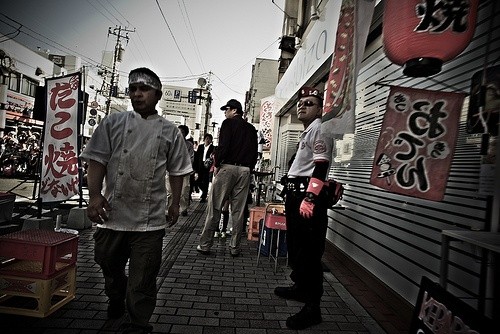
[274,87,333,329]
[79,68,193,331]
[197,99,258,256]
[187,137,197,206]
[165,125,194,221]
[214,194,229,238]
[197,134,216,203]
[242,202,248,236]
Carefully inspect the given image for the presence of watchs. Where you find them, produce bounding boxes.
[305,192,317,202]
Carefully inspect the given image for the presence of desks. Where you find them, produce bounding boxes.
[440,230,500,322]
[252,171,275,207]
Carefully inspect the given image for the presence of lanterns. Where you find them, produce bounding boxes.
[381,0,477,77]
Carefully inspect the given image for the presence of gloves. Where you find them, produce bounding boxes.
[299,193,316,219]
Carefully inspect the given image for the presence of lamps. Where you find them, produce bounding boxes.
[310,0,318,20]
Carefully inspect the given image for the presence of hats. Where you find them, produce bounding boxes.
[220,99,242,111]
[298,86,325,101]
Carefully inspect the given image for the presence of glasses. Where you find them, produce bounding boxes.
[297,101,318,107]
[128,84,154,92]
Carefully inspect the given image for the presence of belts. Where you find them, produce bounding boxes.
[220,161,250,167]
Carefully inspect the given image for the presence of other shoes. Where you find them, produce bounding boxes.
[286,304,322,330]
[221,232,226,238]
[274,284,309,302]
[107,296,125,319]
[200,198,206,203]
[230,250,240,257]
[181,210,188,216]
[214,232,219,238]
[196,244,211,255]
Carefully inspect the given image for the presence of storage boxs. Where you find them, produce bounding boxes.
[247,207,266,241]
[0,229,80,318]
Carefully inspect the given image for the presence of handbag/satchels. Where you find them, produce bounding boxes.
[325,179,344,207]
